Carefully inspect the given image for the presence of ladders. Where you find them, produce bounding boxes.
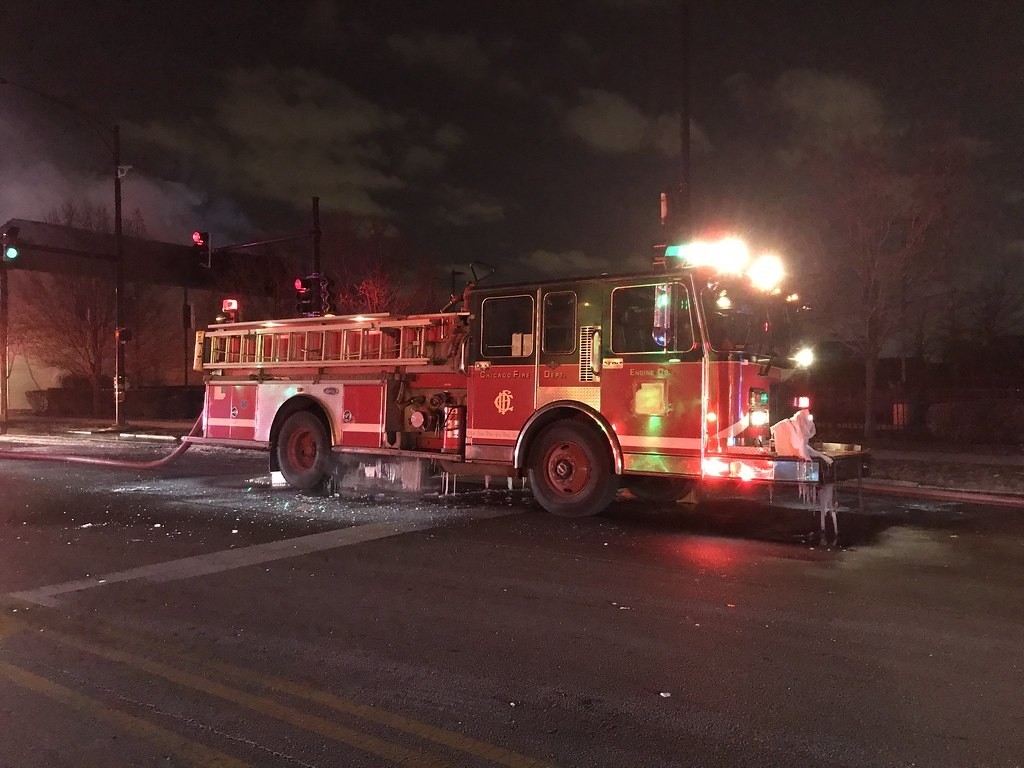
[193,318,433,372]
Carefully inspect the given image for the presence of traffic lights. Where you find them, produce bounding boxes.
[116,330,131,343]
[651,239,674,270]
[191,232,212,268]
[3,227,21,262]
[295,278,312,314]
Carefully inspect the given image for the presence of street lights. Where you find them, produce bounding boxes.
[451,269,465,312]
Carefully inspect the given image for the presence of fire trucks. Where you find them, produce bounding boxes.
[181,231,872,520]
